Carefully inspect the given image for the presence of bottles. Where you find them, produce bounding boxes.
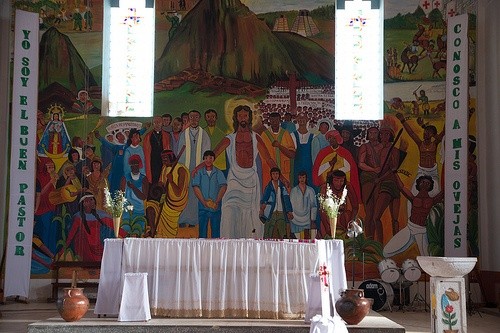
[335,289,370,325]
[57,287,89,322]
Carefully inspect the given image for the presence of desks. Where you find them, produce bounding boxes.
[94,238,348,323]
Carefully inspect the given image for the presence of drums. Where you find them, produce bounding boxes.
[402,259,421,282]
[378,258,399,283]
[358,279,395,312]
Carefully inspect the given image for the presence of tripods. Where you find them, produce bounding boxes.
[409,280,430,312]
[465,272,482,318]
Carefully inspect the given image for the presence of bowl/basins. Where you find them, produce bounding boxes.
[415,256,477,276]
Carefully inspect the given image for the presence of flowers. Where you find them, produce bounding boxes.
[103,178,134,216]
[317,183,348,218]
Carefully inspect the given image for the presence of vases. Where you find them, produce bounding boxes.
[113,214,121,238]
[335,289,370,324]
[330,217,337,238]
[56,288,89,322]
